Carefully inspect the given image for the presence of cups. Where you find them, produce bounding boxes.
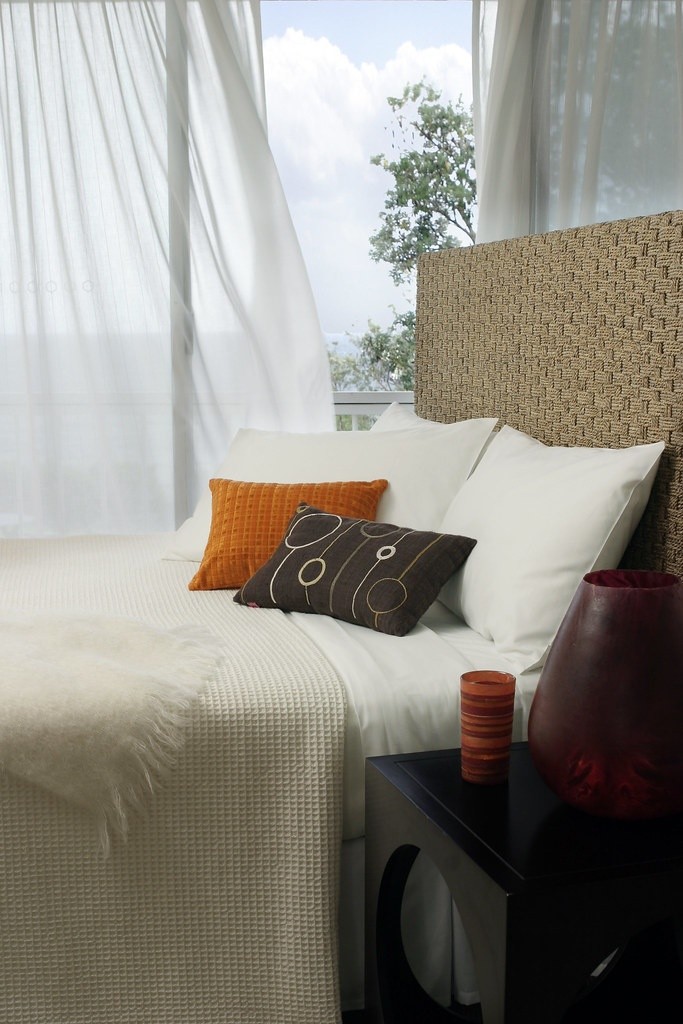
[459,671,516,787]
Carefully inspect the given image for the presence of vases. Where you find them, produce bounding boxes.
[528,567,683,817]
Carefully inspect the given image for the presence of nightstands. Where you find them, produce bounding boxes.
[364,742,683,1024]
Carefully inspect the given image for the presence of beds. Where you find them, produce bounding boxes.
[1,210,683,1024]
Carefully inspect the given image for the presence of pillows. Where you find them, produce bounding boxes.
[368,400,497,468]
[159,418,500,561]
[435,424,665,674]
[233,501,477,636]
[187,479,387,591]
